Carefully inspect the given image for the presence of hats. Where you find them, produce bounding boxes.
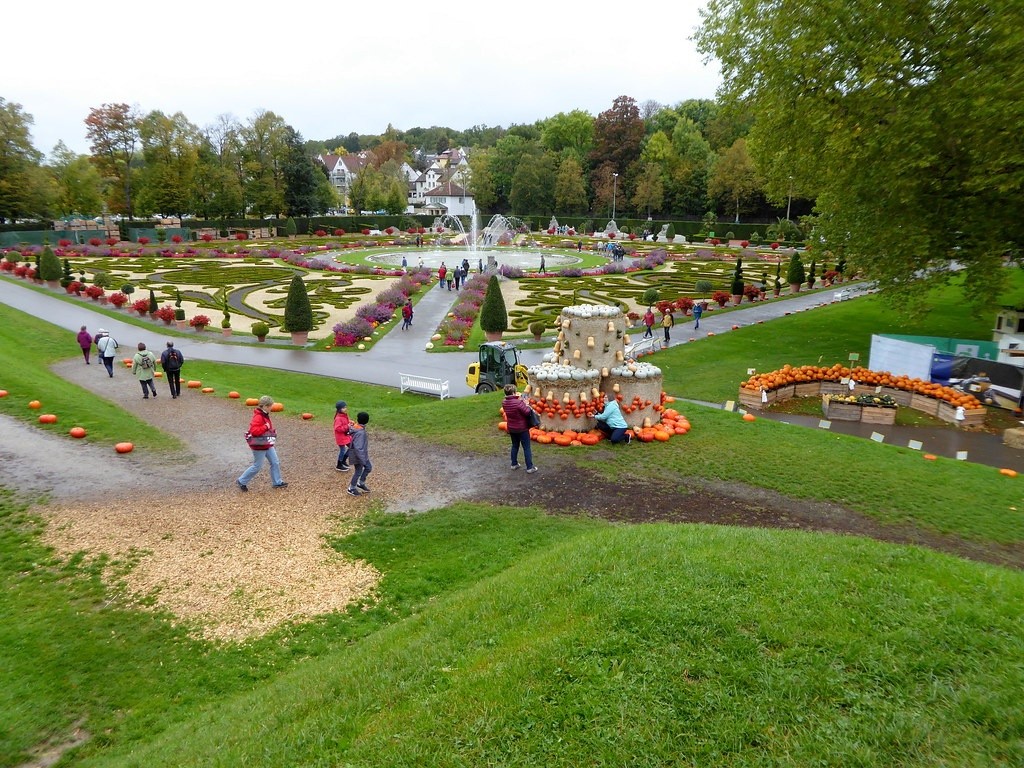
[336,401,346,410]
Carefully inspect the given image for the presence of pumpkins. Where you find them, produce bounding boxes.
[202,388,214,393]
[187,380,202,388]
[245,398,259,406]
[0,389,8,397]
[740,363,983,410]
[303,413,313,419]
[923,454,936,459]
[743,413,754,421]
[498,304,690,446]
[1014,407,1022,412]
[1000,468,1017,476]
[156,359,161,363]
[70,427,85,438]
[270,402,284,412]
[685,283,873,342]
[155,372,162,377]
[326,313,464,350]
[179,378,185,383]
[123,358,133,367]
[28,400,40,409]
[229,391,240,398]
[39,414,57,423]
[115,442,133,453]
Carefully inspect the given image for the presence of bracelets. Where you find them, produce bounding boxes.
[593,415,595,418]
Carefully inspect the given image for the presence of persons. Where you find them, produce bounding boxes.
[77,326,184,400]
[401,233,492,330]
[587,393,632,445]
[502,384,537,473]
[236,396,288,492]
[539,225,655,275]
[334,401,373,495]
[642,302,702,342]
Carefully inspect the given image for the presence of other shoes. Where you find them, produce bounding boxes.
[143,396,148,398]
[511,464,521,469]
[664,339,669,342]
[336,465,349,471]
[343,461,352,468]
[625,433,631,444]
[236,479,248,492]
[173,394,176,399]
[527,467,538,474]
[272,482,289,488]
[110,373,113,377]
[153,391,156,397]
[643,336,646,338]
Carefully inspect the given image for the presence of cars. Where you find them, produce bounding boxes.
[327,207,387,215]
[263,214,277,220]
[60,213,193,224]
[0,216,42,225]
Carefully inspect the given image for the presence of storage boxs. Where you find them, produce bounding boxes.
[739,381,988,427]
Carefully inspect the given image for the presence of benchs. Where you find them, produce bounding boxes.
[398,372,450,400]
[625,337,654,359]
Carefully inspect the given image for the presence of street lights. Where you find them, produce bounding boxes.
[611,172,619,219]
[787,176,794,220]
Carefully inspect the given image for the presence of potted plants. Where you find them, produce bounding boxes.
[609,221,695,245]
[386,227,444,239]
[731,258,781,305]
[316,228,370,239]
[530,323,545,341]
[1,235,268,343]
[676,297,694,314]
[284,275,313,346]
[286,216,297,240]
[480,276,508,342]
[656,301,676,320]
[710,238,801,251]
[712,290,730,307]
[626,313,640,326]
[547,223,594,238]
[694,280,711,310]
[787,251,857,293]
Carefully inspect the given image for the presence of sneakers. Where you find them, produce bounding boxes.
[357,484,370,493]
[347,487,361,496]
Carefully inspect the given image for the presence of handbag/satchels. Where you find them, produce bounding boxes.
[527,410,540,429]
[452,282,455,288]
[99,352,103,358]
[243,414,277,445]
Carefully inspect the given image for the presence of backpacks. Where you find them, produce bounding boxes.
[167,349,181,369]
[139,353,152,369]
